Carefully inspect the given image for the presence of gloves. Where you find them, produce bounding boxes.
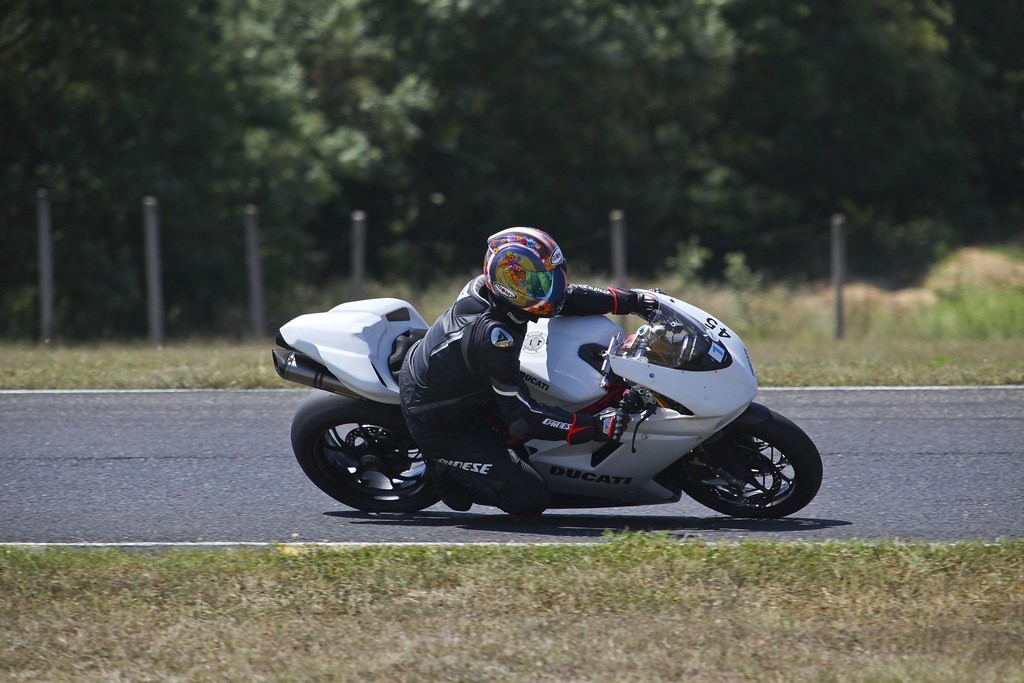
[567,412,632,446]
[608,286,667,322]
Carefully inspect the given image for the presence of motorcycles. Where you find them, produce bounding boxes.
[272,289,824,520]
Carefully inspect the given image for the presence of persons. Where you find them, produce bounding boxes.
[399,228,658,517]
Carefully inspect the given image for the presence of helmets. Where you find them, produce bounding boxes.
[482,226,569,318]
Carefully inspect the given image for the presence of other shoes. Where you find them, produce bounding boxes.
[433,475,473,511]
